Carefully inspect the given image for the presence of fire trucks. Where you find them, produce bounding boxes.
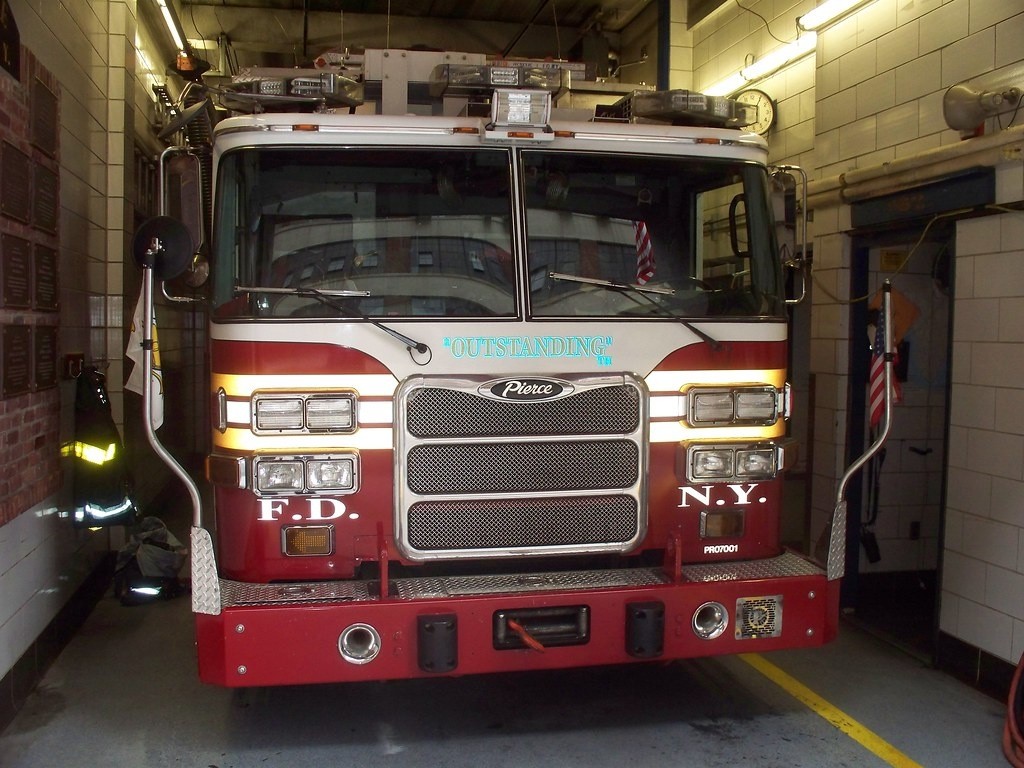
[132,47,894,687]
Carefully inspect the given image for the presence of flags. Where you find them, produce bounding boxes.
[868,300,902,426]
[634,220,656,285]
[125,279,164,429]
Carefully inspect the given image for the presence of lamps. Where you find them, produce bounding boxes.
[795,0,876,34]
[702,30,817,97]
[943,83,1020,132]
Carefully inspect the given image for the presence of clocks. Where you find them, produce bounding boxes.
[727,89,777,135]
